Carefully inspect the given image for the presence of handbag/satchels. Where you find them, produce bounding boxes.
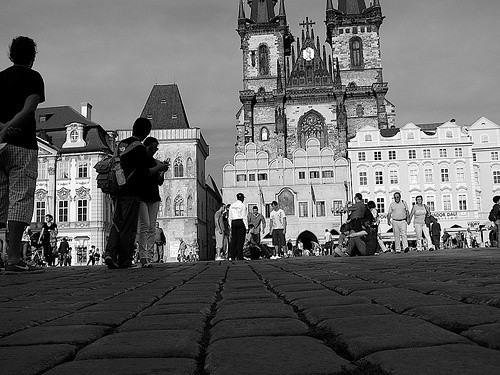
[248,223,256,229]
[363,204,374,223]
[423,204,433,223]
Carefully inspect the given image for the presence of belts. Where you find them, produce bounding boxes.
[393,218,407,221]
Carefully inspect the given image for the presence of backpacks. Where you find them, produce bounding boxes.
[93,140,145,194]
[488,206,498,222]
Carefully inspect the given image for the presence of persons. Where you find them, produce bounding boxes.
[86,246,100,266]
[323,229,333,255]
[387,192,441,254]
[58,237,72,267]
[304,243,328,257]
[333,193,395,257]
[488,196,500,248]
[228,192,249,260]
[296,240,303,257]
[442,228,480,249]
[0,35,44,273]
[247,205,266,243]
[20,214,57,269]
[101,117,170,272]
[214,203,231,261]
[177,238,198,262]
[287,239,294,258]
[243,225,282,260]
[268,201,288,259]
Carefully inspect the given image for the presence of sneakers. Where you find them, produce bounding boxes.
[4,257,46,274]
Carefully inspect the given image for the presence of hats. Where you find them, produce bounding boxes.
[237,193,245,198]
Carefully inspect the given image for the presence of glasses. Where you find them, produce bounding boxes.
[147,146,159,152]
[394,197,399,198]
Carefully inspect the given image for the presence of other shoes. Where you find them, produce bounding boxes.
[335,247,351,257]
[284,254,289,258]
[395,247,409,254]
[429,248,433,251]
[270,255,281,259]
[216,256,225,261]
[104,256,118,270]
[131,258,154,267]
